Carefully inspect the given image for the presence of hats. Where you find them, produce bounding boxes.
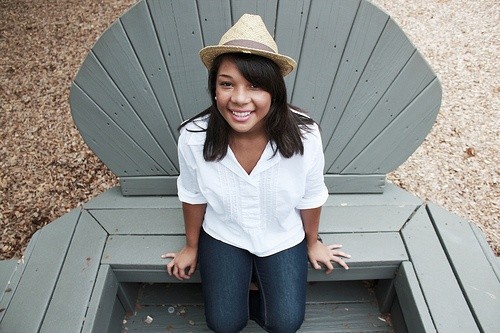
[199,13,297,76]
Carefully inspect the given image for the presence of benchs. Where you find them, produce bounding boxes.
[389,201,500,333]
[0,207,126,333]
[83,183,423,314]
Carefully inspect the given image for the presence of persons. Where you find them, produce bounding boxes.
[161,13,352,333]
